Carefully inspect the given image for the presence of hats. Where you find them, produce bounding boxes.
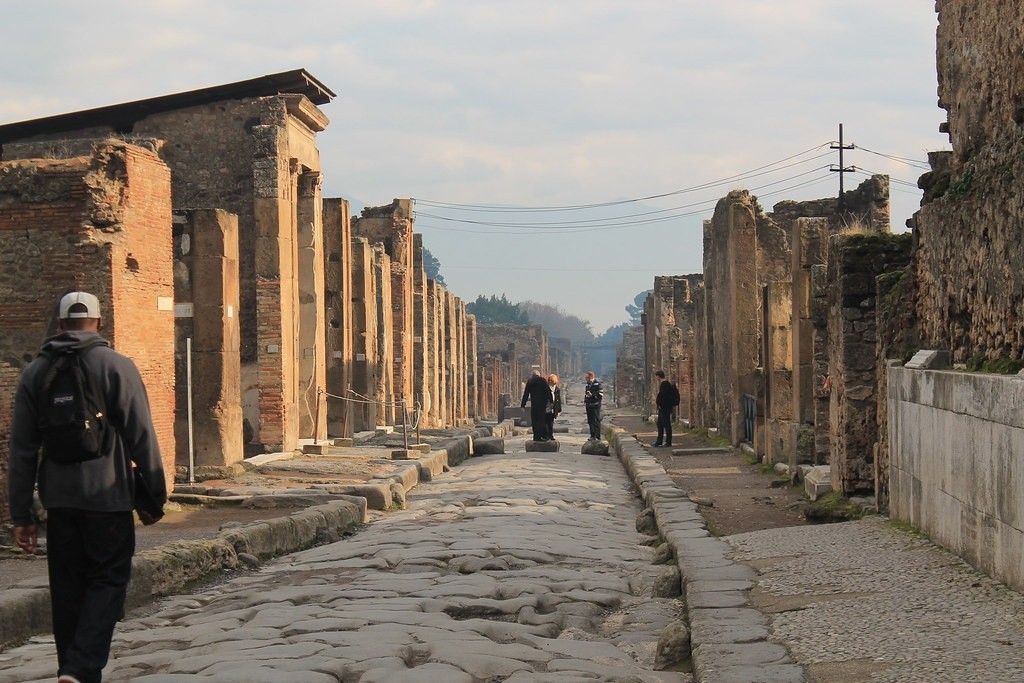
[60,292,101,318]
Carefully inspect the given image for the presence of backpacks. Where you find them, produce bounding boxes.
[36,343,111,464]
[664,380,680,406]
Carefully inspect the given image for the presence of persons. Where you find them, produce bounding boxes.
[652,370,674,447]
[8,291,167,683]
[584,372,603,442]
[521,369,553,441]
[544,373,562,439]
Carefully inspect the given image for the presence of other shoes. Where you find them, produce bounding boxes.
[659,442,670,447]
[587,437,596,440]
[653,443,659,447]
[58,672,82,683]
